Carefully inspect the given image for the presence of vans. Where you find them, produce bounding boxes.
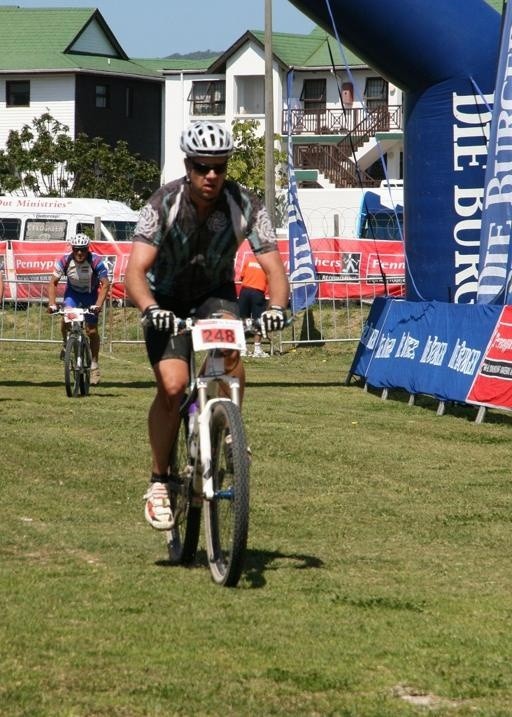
[0,195,140,248]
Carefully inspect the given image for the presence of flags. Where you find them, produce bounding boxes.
[287,109,322,318]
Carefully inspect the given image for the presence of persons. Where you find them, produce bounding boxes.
[45,232,110,386]
[127,117,290,531]
[236,253,271,358]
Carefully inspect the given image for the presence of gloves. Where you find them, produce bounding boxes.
[90,305,100,313]
[143,304,178,337]
[50,304,58,312]
[260,305,286,339]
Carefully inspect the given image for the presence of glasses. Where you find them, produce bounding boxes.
[74,247,86,252]
[187,160,227,173]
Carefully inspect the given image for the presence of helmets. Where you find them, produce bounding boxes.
[179,121,234,157]
[71,233,90,245]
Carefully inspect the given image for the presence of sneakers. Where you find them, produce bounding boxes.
[143,482,175,530]
[240,350,269,357]
[90,368,100,385]
[224,435,251,470]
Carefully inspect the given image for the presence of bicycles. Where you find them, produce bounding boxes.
[139,313,292,587]
[46,305,103,397]
